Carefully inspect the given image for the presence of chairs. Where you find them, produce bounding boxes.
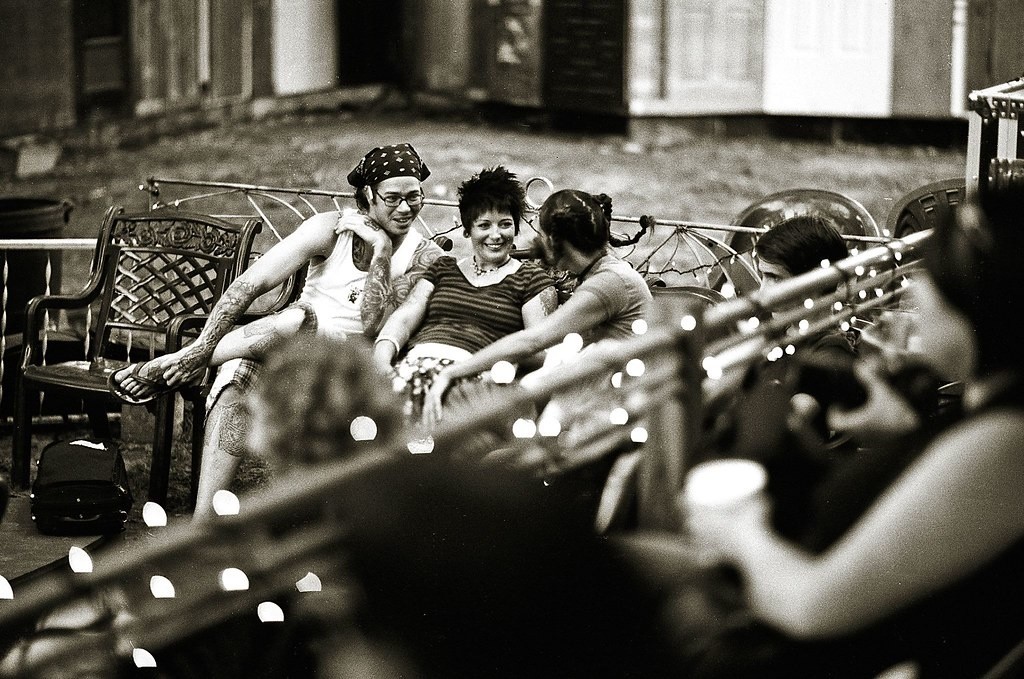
[9,205,262,506]
[703,177,965,298]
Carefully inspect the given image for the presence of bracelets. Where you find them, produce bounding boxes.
[373,335,400,356]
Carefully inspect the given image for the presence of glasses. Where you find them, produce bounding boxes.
[374,187,426,207]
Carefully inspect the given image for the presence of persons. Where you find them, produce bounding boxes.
[676,179,1023,679]
[419,190,653,498]
[370,167,558,467]
[106,144,448,525]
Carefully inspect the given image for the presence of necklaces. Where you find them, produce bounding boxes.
[473,254,511,278]
[577,252,607,283]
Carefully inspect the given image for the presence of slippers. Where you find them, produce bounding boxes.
[106,361,205,405]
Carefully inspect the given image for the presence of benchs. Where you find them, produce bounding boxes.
[149,245,732,517]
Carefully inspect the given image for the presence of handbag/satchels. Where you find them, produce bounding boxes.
[31,439,133,534]
